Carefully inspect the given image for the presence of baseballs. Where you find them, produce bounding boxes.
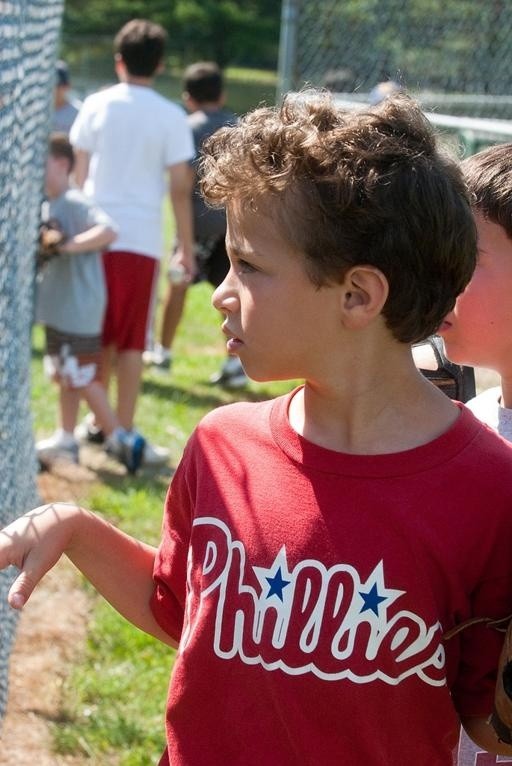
[165,264,187,285]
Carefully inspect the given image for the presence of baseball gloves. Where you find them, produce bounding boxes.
[37,218,67,258]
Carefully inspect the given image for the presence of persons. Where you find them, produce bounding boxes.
[35,133,147,475]
[433,136,512,443]
[53,62,79,136]
[144,64,250,391]
[0,82,512,766]
[67,18,196,468]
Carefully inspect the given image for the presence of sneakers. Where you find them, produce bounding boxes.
[35,440,80,464]
[113,430,170,475]
[212,359,247,386]
[75,422,103,442]
[145,353,170,368]
